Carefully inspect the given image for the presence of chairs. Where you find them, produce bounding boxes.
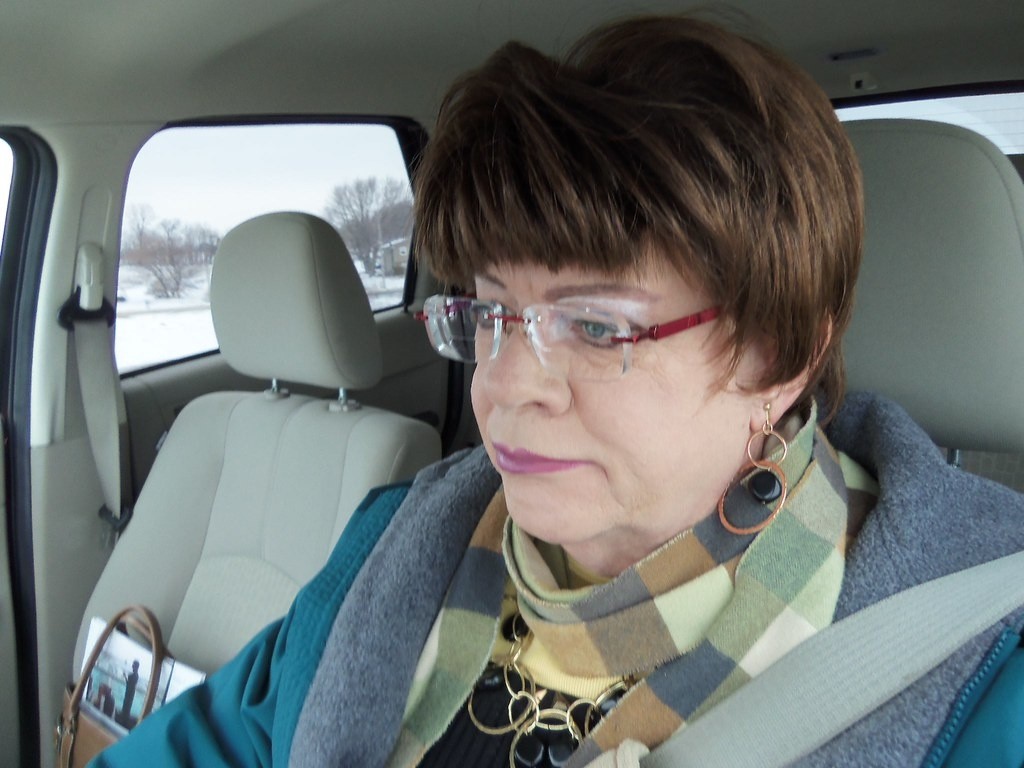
[69,211,444,691]
[831,119,1023,475]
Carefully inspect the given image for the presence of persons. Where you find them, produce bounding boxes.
[121,660,140,717]
[83,15,1024,768]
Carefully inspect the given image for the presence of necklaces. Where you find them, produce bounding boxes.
[466,593,656,768]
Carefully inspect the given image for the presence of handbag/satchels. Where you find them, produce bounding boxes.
[54,605,173,768]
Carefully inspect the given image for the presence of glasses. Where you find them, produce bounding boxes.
[412,295,727,380]
